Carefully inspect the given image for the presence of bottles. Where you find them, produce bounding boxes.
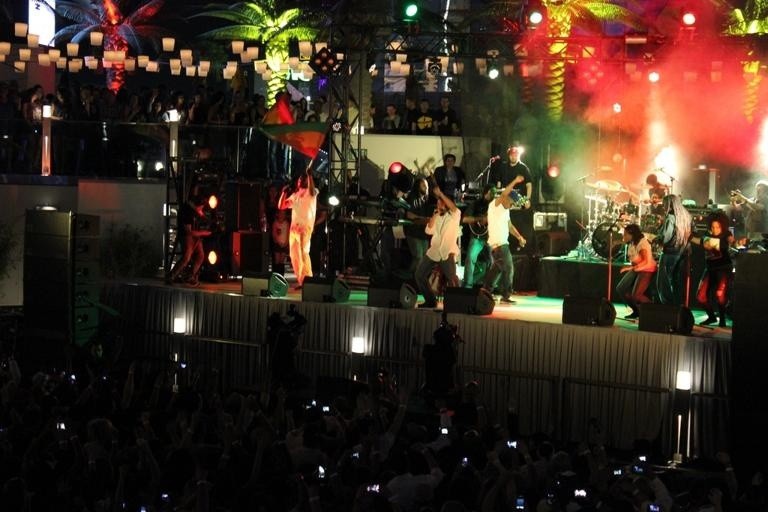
[578,241,591,261]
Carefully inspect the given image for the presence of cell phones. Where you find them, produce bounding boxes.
[516,495,525,510]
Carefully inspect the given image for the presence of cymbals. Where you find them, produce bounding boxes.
[586,184,628,193]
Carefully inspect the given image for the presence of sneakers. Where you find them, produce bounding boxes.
[493,297,516,305]
[418,302,438,308]
[625,312,640,320]
[700,317,726,328]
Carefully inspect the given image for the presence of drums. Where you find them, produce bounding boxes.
[614,204,640,227]
[593,220,626,258]
[602,203,619,223]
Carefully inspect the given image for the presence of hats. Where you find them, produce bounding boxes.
[509,190,520,203]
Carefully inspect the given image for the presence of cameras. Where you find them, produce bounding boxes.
[287,304,296,316]
[440,427,467,468]
[507,440,517,449]
[305,399,330,413]
[318,451,381,494]
[54,351,187,394]
[56,422,65,431]
[122,493,169,512]
[573,456,660,512]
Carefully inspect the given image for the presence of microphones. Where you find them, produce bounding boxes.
[649,168,663,171]
[435,208,446,214]
[492,155,501,163]
[284,183,292,192]
[692,215,703,221]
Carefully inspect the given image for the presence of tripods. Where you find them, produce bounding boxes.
[564,178,600,262]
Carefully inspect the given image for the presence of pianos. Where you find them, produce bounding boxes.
[465,189,526,203]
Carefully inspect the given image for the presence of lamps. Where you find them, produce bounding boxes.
[0,0,542,81]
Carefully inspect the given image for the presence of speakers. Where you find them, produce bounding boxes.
[638,303,693,334]
[242,272,289,297]
[24,208,98,363]
[368,283,417,309]
[729,253,768,489]
[302,276,351,303]
[445,287,495,315]
[563,297,616,327]
